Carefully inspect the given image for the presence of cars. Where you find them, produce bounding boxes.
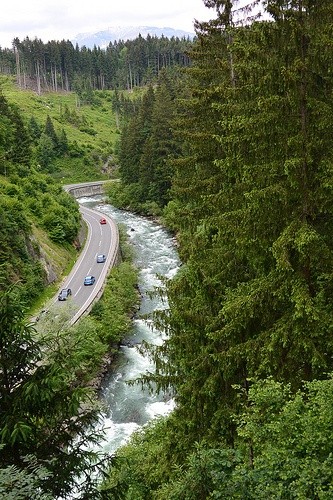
[96,254,106,263]
[36,311,50,321]
[100,218,106,224]
[84,276,96,286]
[58,288,72,301]
